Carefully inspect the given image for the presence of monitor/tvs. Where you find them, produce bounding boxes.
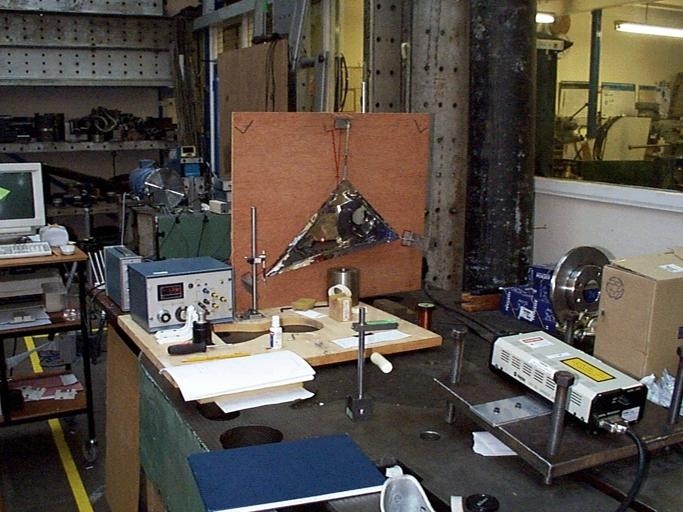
[0,162,46,245]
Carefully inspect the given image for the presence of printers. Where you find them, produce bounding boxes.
[0,265,67,331]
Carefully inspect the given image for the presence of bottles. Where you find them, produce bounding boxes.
[327,266,359,308]
[269,316,283,350]
[418,302,434,329]
[193,310,214,348]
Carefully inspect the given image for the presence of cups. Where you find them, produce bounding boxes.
[59,241,76,256]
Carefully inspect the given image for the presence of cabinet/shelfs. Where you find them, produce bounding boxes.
[1,83,180,245]
[3,252,98,455]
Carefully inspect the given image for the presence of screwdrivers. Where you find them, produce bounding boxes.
[168,343,233,355]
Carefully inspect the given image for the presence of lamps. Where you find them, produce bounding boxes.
[614,3,682,35]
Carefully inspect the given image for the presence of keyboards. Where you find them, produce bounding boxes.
[0,241,52,259]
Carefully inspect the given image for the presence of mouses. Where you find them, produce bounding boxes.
[60,244,75,255]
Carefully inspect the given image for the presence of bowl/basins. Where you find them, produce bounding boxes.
[62,309,80,321]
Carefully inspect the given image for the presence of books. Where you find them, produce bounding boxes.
[188,434,386,512]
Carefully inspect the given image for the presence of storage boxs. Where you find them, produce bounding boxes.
[594,249,683,382]
